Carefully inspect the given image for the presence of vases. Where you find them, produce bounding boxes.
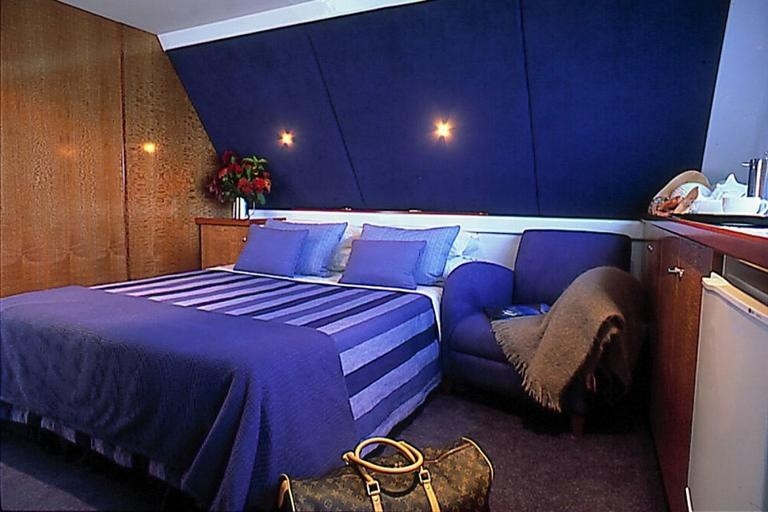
[232,197,255,219]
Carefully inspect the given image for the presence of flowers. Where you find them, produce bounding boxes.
[209,150,271,204]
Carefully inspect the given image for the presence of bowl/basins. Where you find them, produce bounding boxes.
[694,198,724,214]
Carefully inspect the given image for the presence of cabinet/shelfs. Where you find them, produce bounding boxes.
[643,220,662,456]
[660,227,724,512]
[0,0,129,299]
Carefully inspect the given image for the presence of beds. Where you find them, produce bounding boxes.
[0,260,510,512]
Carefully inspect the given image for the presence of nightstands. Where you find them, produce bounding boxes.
[194,215,287,271]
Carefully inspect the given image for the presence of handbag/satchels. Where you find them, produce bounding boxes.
[276,434,496,512]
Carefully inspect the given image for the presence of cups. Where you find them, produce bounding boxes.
[722,196,768,215]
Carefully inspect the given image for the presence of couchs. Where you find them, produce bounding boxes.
[440,228,635,436]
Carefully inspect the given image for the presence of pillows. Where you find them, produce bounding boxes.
[337,239,427,290]
[233,223,307,278]
[360,224,461,286]
[265,217,348,278]
